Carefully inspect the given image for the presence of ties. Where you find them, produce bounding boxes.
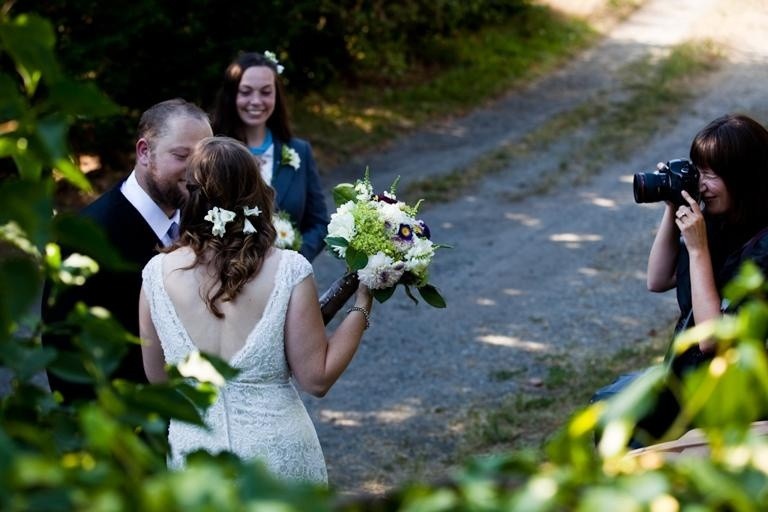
[167,222,179,242]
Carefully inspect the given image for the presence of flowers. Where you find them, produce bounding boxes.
[280,144,302,170]
[268,208,303,250]
[266,50,284,73]
[204,204,262,237]
[323,165,453,309]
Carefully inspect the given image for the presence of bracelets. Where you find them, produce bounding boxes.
[347,306,369,329]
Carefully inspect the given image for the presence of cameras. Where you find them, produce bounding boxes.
[630,156,701,218]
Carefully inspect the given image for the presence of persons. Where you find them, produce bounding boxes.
[41,97,214,407]
[647,113,768,449]
[210,50,331,263]
[138,136,373,490]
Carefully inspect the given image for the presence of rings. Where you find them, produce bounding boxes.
[679,212,686,219]
